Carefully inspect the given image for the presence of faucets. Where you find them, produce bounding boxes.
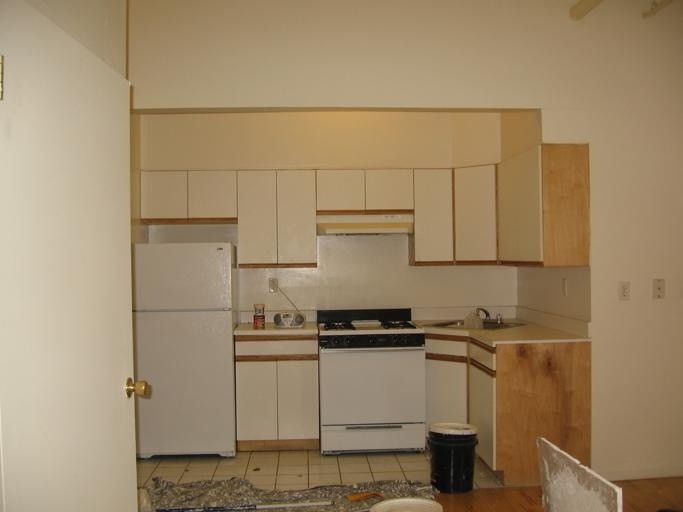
[472,306,492,320]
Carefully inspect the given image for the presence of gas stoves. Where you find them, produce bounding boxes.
[317,317,425,336]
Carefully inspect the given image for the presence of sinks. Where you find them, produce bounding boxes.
[432,317,527,330]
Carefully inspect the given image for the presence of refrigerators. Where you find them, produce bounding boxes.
[132,241,240,460]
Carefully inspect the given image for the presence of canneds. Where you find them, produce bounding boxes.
[254,315,266,329]
[254,303,265,315]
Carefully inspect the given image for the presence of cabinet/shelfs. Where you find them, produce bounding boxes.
[425,334,592,486]
[140,107,591,268]
[235,336,319,451]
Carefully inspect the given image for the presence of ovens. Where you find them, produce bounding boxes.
[317,332,426,456]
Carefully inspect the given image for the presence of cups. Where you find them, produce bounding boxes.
[253,303,265,316]
[253,314,265,329]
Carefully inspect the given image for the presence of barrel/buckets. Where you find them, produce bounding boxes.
[370,497,445,512]
[463,311,484,328]
[427,424,479,493]
